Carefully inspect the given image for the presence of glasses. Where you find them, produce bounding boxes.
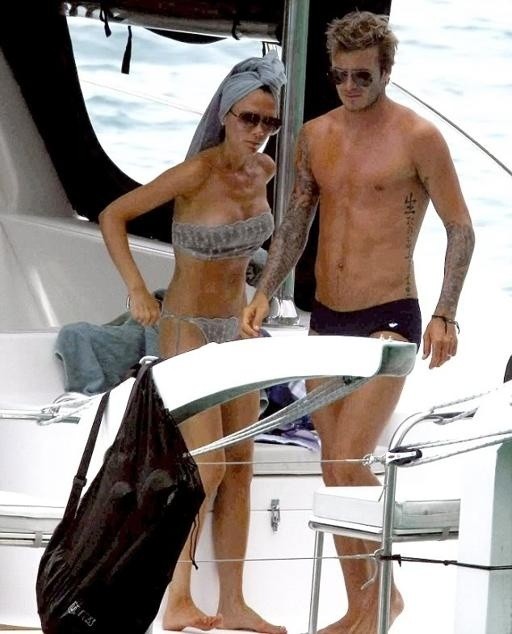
[329,69,376,89]
[228,108,282,137]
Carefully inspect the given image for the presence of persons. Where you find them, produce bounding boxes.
[100,53,287,632]
[237,8,476,633]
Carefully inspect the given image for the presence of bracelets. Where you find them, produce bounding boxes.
[431,315,460,336]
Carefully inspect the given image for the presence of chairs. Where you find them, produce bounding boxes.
[300,381,512,634]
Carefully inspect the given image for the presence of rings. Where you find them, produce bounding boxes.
[447,354,452,357]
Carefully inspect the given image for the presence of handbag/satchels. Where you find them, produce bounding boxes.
[36,370,204,633]
